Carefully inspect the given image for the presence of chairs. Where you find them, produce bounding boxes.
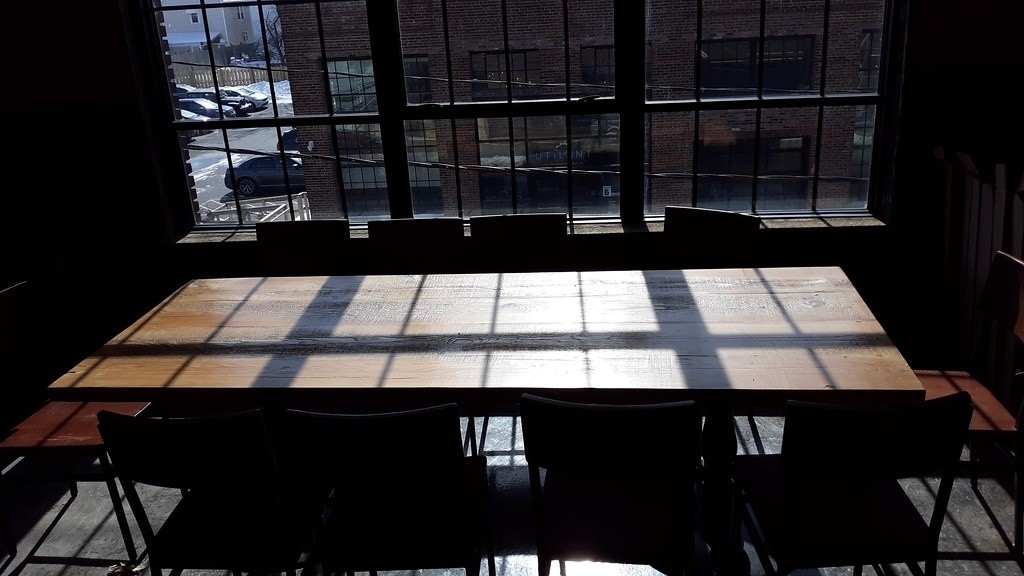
[0,206,1024,576]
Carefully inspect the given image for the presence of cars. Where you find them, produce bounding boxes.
[278,128,301,152]
[181,109,214,135]
[224,152,310,197]
[221,87,269,111]
[176,84,196,97]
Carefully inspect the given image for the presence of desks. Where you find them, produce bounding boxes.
[47,265,925,439]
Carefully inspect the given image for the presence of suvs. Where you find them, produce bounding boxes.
[179,98,236,120]
[182,89,252,116]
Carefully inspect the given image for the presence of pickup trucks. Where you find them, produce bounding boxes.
[231,57,241,64]
